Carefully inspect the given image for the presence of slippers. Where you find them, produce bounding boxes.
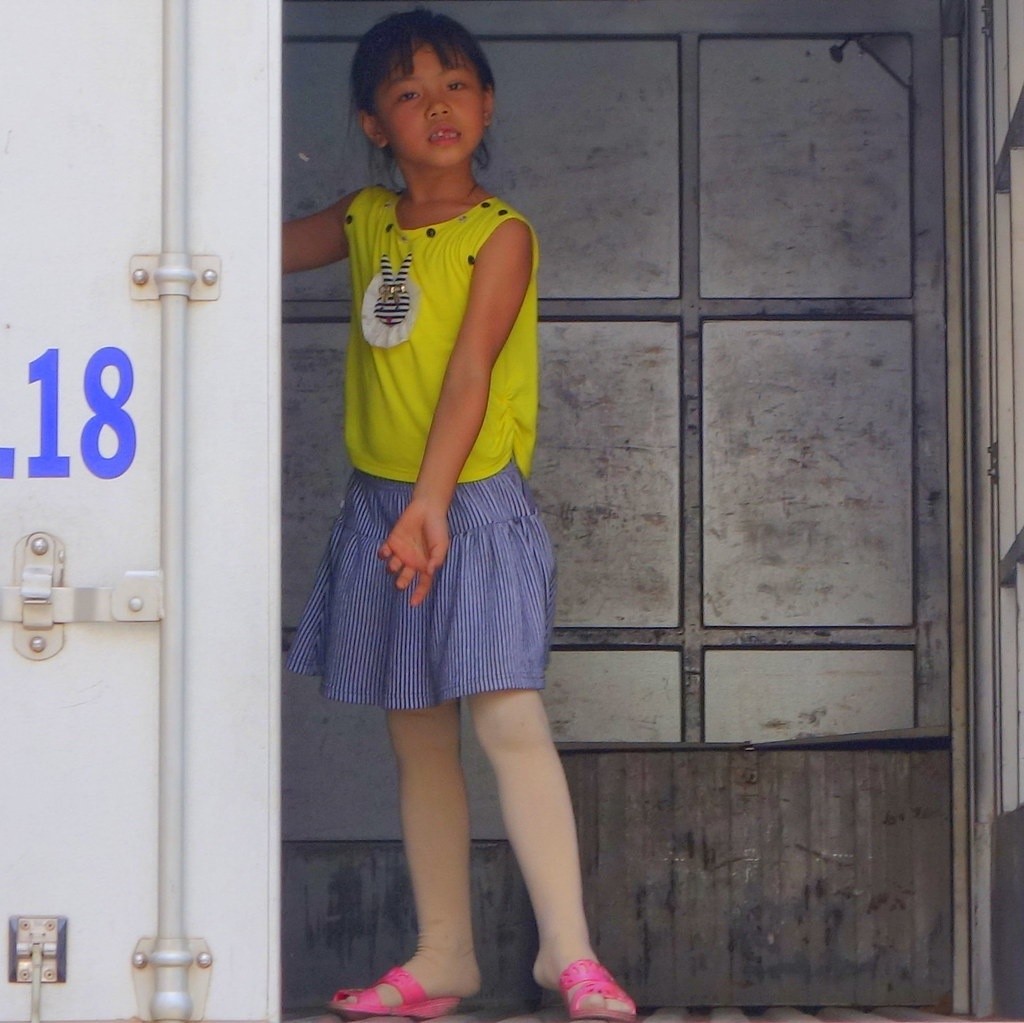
[328,967,462,1018]
[557,959,638,1021]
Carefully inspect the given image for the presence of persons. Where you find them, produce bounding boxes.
[283,6,643,1018]
